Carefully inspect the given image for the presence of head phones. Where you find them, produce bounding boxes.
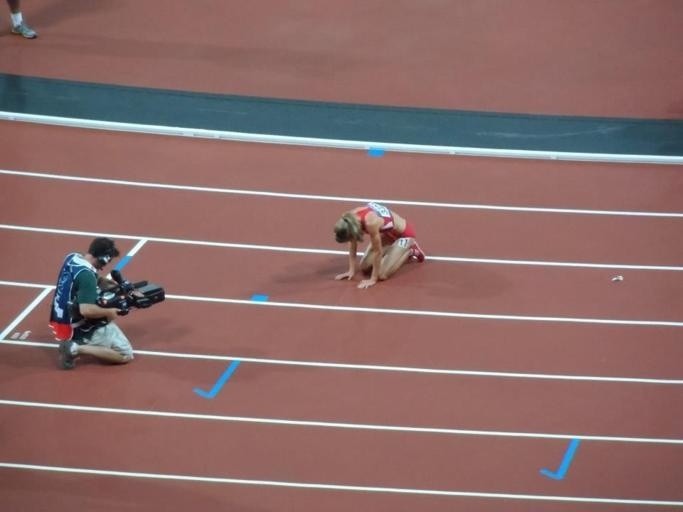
[98,244,114,265]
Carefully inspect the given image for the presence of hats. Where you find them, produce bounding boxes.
[86,237,120,259]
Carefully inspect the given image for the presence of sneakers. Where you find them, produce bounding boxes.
[10,20,37,39]
[408,240,426,263]
[57,339,78,371]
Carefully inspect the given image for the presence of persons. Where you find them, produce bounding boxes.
[45,238,136,369]
[4,0,37,39]
[331,202,427,288]
